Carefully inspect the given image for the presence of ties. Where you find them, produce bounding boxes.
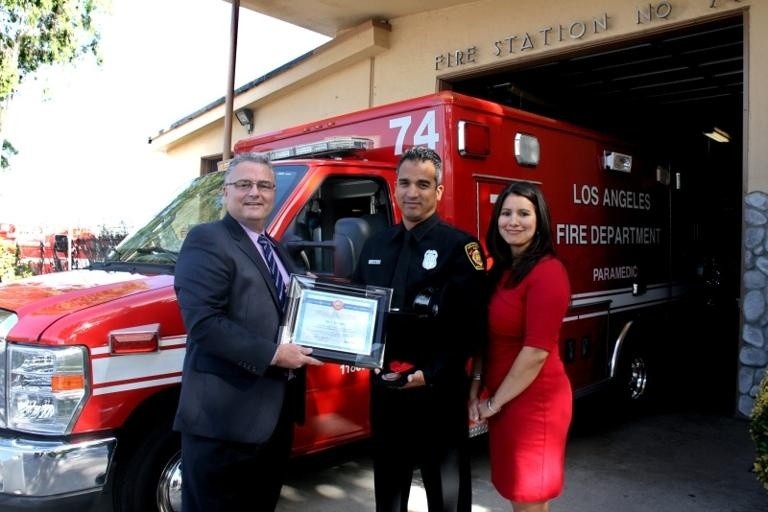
[258,236,289,313]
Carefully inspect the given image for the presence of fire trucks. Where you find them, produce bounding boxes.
[0,87,733,512]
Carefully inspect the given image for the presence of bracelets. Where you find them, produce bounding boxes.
[472,372,481,381]
[487,400,496,414]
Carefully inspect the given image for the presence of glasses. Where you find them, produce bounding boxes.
[225,179,276,191]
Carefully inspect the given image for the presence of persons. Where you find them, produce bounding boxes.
[467,181,574,511]
[350,147,486,512]
[172,153,325,512]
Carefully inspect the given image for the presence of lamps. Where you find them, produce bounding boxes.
[234,108,254,134]
